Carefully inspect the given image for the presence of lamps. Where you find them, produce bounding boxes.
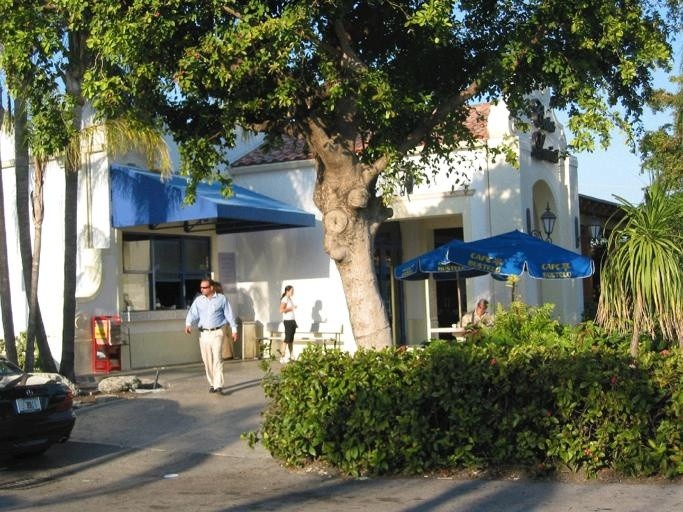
[527,201,556,245]
[575,208,602,248]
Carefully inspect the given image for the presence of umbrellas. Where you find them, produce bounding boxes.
[442,220,596,311]
[393,236,488,326]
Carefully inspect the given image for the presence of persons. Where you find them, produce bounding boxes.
[182,277,237,396]
[450,296,495,345]
[276,284,299,364]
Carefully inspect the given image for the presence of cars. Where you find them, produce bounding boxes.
[0,355,75,460]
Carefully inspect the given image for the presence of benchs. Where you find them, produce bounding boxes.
[256,322,343,358]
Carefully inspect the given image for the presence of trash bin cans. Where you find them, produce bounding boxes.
[232,315,257,360]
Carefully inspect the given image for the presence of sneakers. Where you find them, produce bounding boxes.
[208,386,224,396]
[280,356,297,364]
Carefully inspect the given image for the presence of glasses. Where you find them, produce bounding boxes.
[200,286,210,289]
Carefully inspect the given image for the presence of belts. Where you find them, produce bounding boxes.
[202,327,221,331]
